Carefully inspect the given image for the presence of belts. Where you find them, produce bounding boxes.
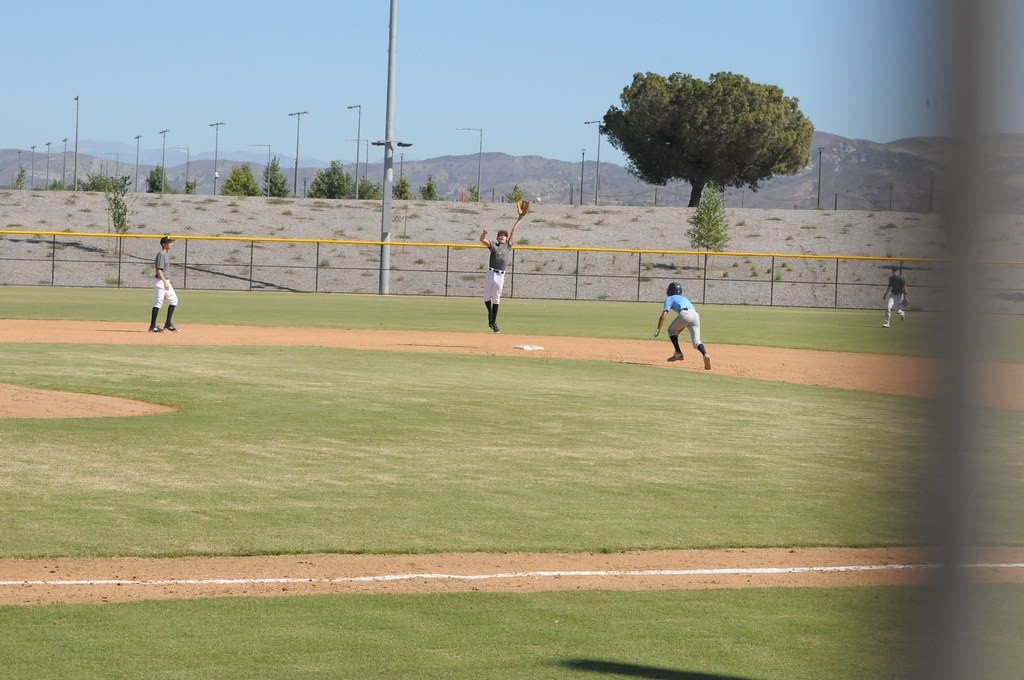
[679,308,688,311]
[489,269,504,273]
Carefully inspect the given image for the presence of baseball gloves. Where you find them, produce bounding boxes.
[517,199,533,215]
[899,299,909,307]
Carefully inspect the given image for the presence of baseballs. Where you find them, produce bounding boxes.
[536,197,541,202]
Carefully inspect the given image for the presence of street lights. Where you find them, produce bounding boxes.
[74,95,80,190]
[288,112,311,197]
[159,129,170,193]
[347,105,362,200]
[580,148,587,205]
[62,138,70,190]
[584,120,601,205]
[46,142,52,191]
[817,145,824,206]
[135,135,142,192]
[209,122,226,195]
[30,145,36,190]
[17,150,23,175]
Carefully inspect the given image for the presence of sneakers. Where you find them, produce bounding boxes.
[149,326,163,333]
[165,324,179,331]
[703,353,711,370]
[667,352,684,361]
[489,322,500,332]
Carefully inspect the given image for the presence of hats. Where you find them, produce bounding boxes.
[497,230,508,238]
[891,265,899,270]
[160,237,174,245]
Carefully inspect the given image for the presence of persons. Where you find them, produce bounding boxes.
[149,237,178,333]
[882,266,908,328]
[654,282,711,370]
[480,200,529,334]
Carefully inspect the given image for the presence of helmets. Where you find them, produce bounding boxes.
[666,283,682,296]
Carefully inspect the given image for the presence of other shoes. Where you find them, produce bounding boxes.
[883,323,890,328]
[900,314,904,321]
